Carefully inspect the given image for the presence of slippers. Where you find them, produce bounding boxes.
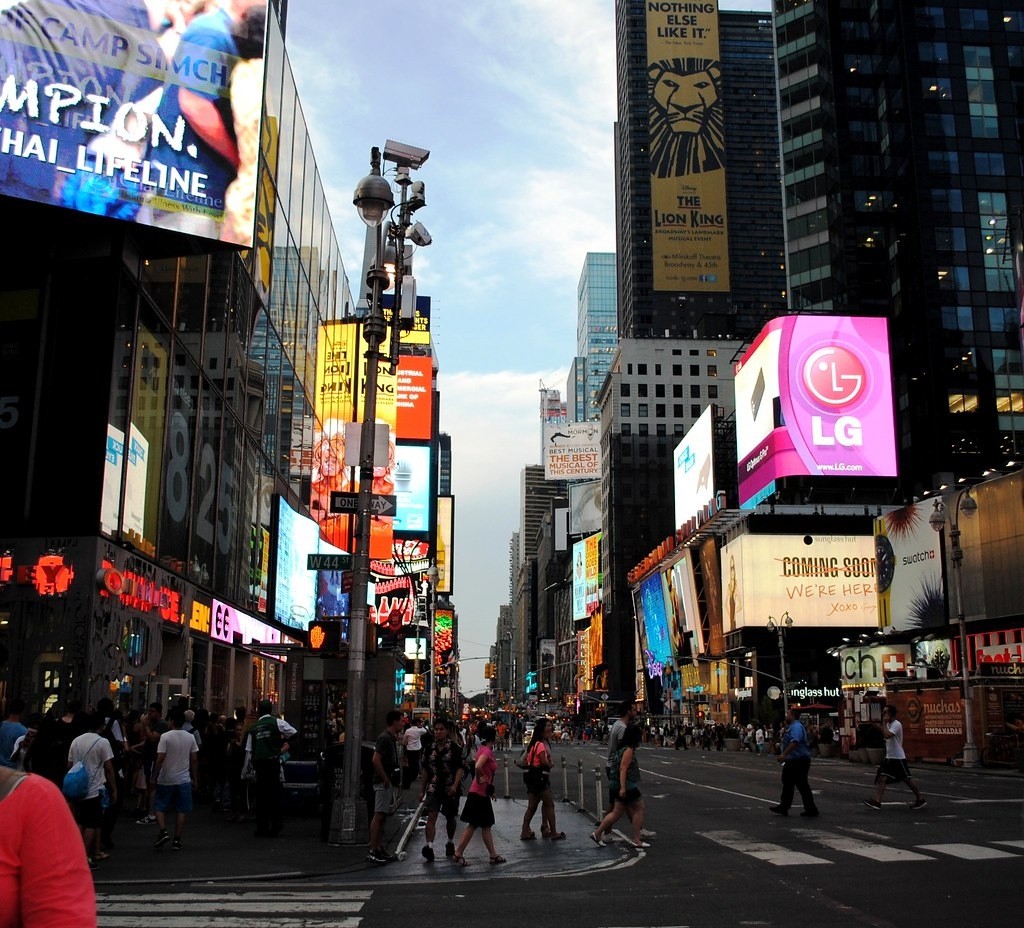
[552,832,565,840]
[521,831,536,840]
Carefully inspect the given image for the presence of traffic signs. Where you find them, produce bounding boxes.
[329,491,397,516]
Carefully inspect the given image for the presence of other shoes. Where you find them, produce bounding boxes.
[422,845,434,862]
[89,860,99,869]
[799,811,819,817]
[541,826,551,837]
[768,805,788,817]
[446,842,455,857]
[590,824,658,847]
[94,851,108,859]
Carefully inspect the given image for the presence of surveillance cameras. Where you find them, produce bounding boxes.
[411,222,432,246]
[383,139,430,167]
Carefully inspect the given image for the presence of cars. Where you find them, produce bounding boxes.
[495,710,537,734]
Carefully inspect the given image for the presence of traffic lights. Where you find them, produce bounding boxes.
[696,710,705,719]
[489,662,498,679]
[308,621,341,653]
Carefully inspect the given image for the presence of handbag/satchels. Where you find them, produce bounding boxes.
[516,748,529,769]
[389,762,401,787]
[485,784,494,796]
[240,762,257,783]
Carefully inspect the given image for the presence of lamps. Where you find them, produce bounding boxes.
[911,636,922,643]
[923,490,958,495]
[957,476,993,483]
[939,484,968,490]
[925,634,935,640]
[191,555,201,581]
[826,646,838,654]
[859,633,868,638]
[200,562,209,585]
[831,645,847,656]
[982,470,1012,476]
[874,631,884,635]
[1005,460,1024,467]
[870,641,879,648]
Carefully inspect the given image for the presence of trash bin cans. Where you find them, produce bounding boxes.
[989,732,1019,771]
[317,746,376,841]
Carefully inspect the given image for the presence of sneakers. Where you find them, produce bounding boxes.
[377,846,393,858]
[153,831,170,847]
[136,815,157,825]
[863,798,882,810]
[172,840,184,849]
[365,848,386,863]
[909,798,927,810]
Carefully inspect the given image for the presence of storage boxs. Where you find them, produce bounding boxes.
[916,667,940,680]
[284,760,319,781]
[282,783,319,817]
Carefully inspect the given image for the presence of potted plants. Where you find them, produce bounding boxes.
[817,723,885,764]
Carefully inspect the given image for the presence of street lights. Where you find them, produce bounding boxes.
[425,557,442,725]
[336,146,396,848]
[766,612,794,721]
[928,485,983,768]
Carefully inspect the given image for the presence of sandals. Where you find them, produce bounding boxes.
[452,854,470,866]
[490,855,506,864]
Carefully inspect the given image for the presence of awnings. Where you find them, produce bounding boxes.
[234,641,301,657]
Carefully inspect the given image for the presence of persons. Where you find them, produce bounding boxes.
[462,706,609,753]
[365,710,482,861]
[520,717,566,840]
[861,704,926,813]
[0,694,301,928]
[598,698,656,843]
[146,0,267,239]
[327,683,347,744]
[799,716,841,758]
[634,717,790,758]
[450,726,507,867]
[590,724,652,848]
[768,708,820,817]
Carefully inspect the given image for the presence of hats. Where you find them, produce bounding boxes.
[259,699,272,712]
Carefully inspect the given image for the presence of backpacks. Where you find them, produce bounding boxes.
[99,718,119,763]
[62,738,102,799]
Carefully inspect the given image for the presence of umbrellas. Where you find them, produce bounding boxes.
[795,701,834,726]
